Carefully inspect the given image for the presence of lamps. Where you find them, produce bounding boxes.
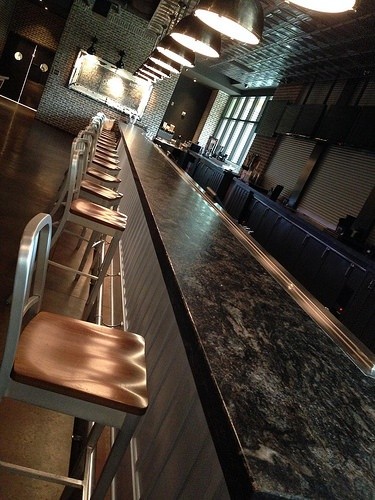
[133,0,361,83]
[115,50,125,68]
[87,35,99,55]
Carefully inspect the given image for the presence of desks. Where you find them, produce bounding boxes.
[111,120,375,500]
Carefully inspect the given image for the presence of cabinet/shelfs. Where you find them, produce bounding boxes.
[225,177,375,348]
[189,159,240,202]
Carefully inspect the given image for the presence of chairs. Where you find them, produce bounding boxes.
[0,112,148,500]
